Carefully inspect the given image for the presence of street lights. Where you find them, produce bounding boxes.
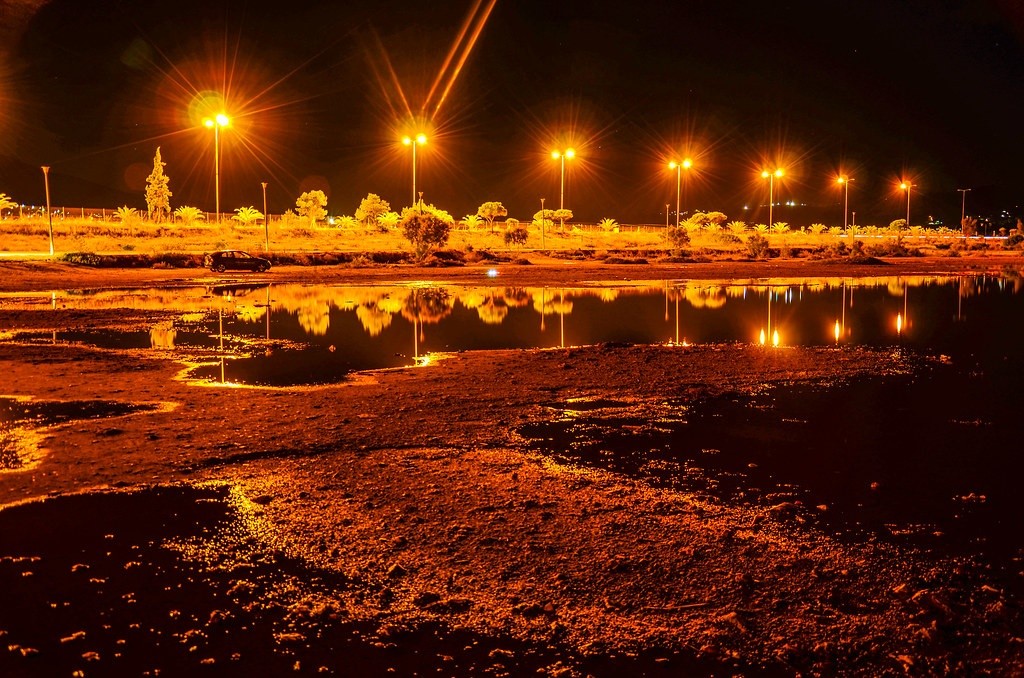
[834,277,851,339]
[958,188,971,233]
[401,133,427,206]
[760,299,779,346]
[838,178,855,232]
[551,148,576,231]
[762,169,783,234]
[204,114,228,223]
[669,158,690,228]
[901,184,917,228]
[896,280,913,331]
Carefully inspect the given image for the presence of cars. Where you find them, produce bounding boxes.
[204,251,272,273]
